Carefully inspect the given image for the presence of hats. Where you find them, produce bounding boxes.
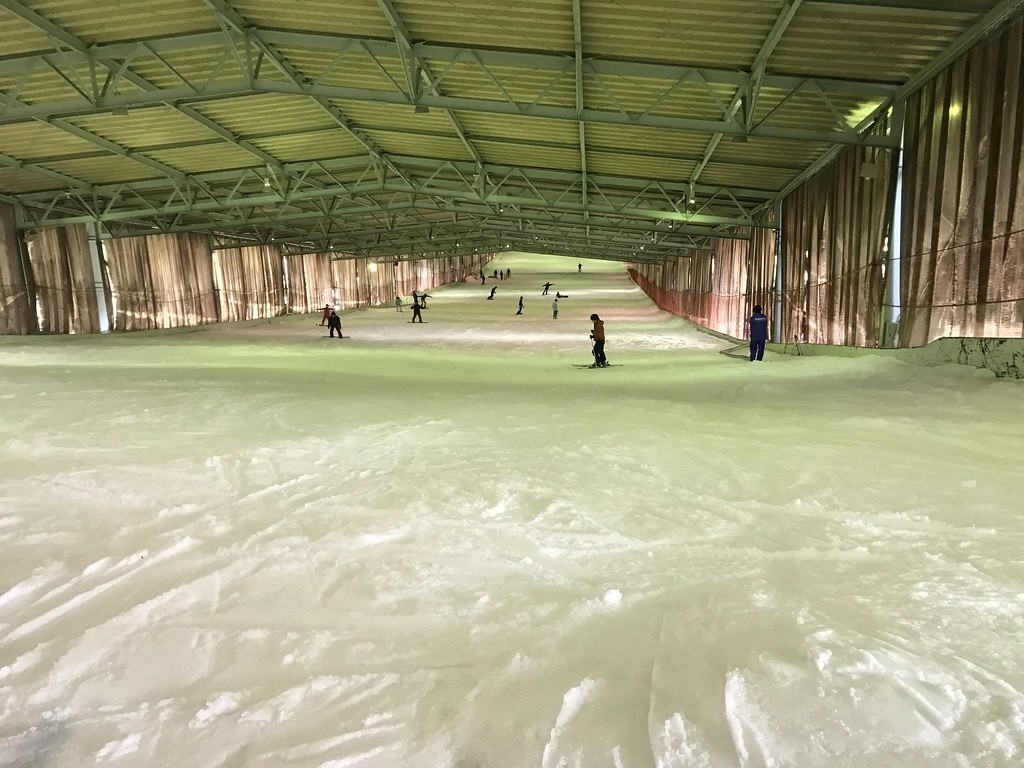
[590,314,599,321]
[753,305,762,313]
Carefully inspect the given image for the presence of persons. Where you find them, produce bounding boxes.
[541,282,554,295]
[589,314,606,367]
[487,285,497,299]
[328,312,342,338]
[395,295,403,312]
[555,292,568,298]
[578,263,582,272]
[316,304,335,325]
[750,306,767,362]
[480,267,511,285]
[411,300,425,323]
[412,290,432,309]
[552,299,558,319]
[517,296,524,315]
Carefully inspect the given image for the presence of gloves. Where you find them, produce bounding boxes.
[591,330,594,333]
[590,335,594,339]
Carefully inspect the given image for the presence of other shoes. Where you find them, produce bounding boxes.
[589,362,602,368]
[600,361,605,367]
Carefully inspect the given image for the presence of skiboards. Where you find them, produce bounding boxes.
[407,321,428,323]
[573,363,623,368]
[323,336,351,338]
[316,323,329,327]
